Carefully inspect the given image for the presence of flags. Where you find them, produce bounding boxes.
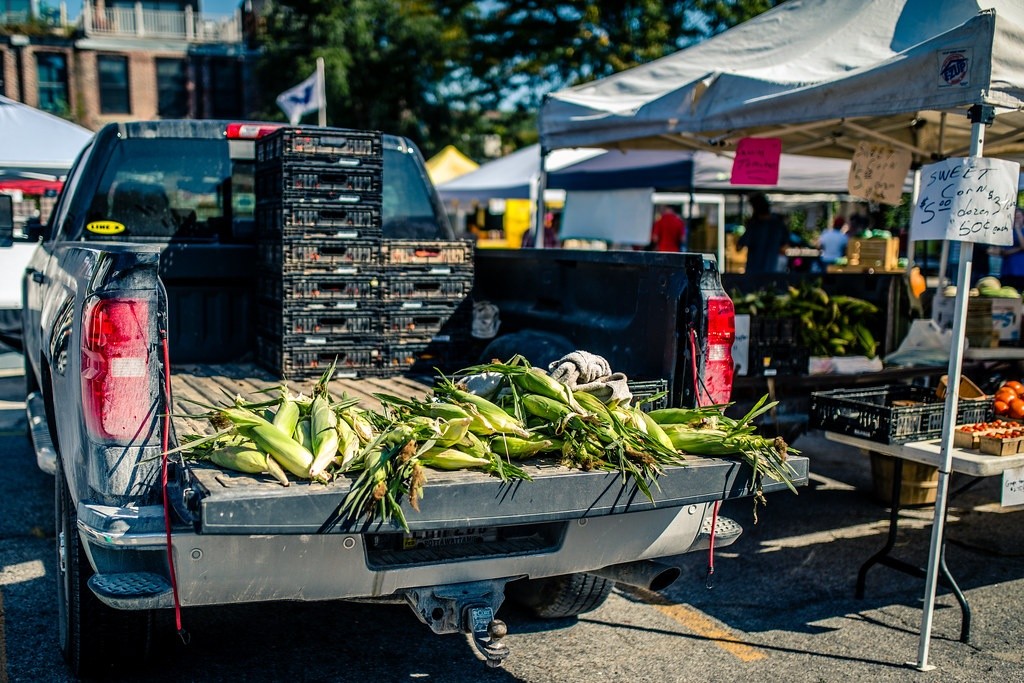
[276,57,327,125]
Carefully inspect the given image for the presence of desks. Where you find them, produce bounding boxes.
[826,428,1024,643]
[966,348,1024,361]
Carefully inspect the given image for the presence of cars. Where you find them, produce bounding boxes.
[178,177,224,215]
[219,185,259,214]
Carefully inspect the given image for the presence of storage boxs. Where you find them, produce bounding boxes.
[965,296,1023,347]
[248,124,475,381]
[747,315,807,376]
[810,384,995,446]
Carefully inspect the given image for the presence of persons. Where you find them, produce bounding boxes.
[811,212,911,292]
[736,195,795,277]
[466,201,506,240]
[725,225,749,275]
[948,207,1024,293]
[521,203,689,254]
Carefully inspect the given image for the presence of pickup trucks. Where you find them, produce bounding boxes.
[1,117,810,674]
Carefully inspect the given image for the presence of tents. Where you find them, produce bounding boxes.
[434,141,727,276]
[535,144,922,269]
[536,0,1024,672]
[0,95,97,183]
[425,146,482,183]
